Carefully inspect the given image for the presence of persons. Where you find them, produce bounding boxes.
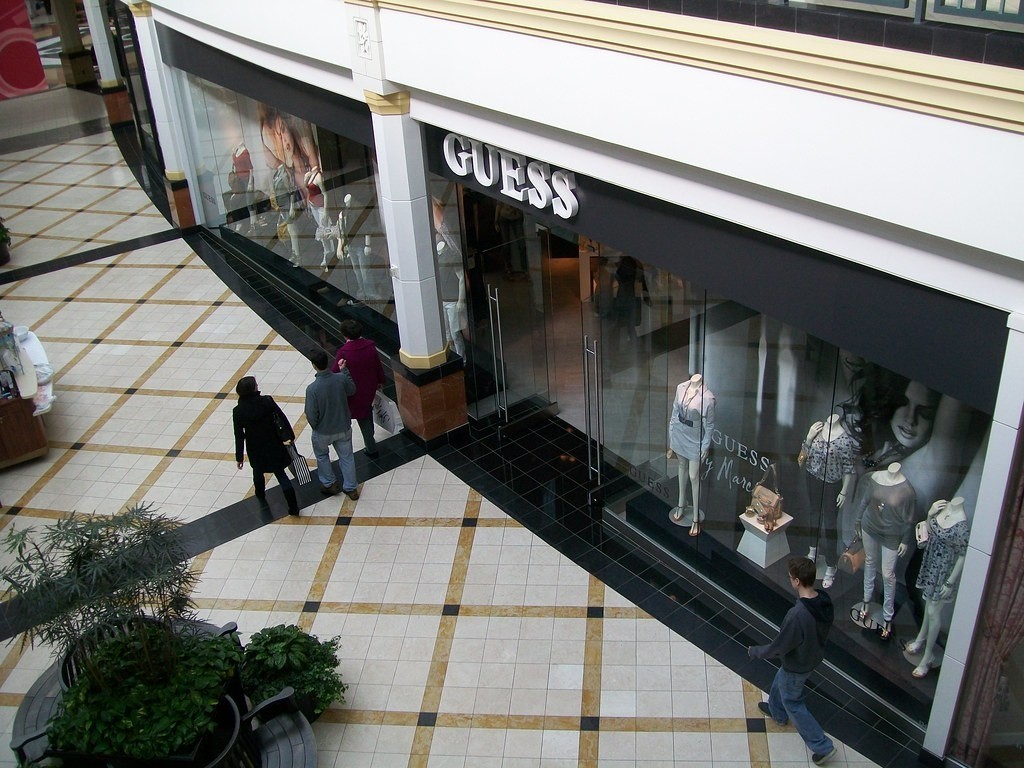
[797,413,859,588]
[854,462,917,639]
[592,256,653,347]
[669,373,718,535]
[304,349,359,501]
[337,194,378,306]
[834,357,973,614]
[232,376,299,516]
[272,164,303,267]
[229,141,257,237]
[472,200,527,280]
[304,166,335,268]
[330,319,386,455]
[906,497,969,680]
[748,555,840,767]
[256,100,321,209]
[436,240,467,367]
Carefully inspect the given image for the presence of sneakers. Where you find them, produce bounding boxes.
[315,472,332,488]
[344,489,358,500]
[367,447,377,454]
[812,745,837,765]
[758,702,786,726]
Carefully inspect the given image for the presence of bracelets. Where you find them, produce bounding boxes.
[365,245,371,247]
[839,492,847,497]
[943,580,955,588]
[248,182,253,185]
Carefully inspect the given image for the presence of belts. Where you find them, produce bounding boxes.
[678,415,693,428]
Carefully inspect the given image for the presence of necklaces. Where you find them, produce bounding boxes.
[862,436,930,471]
[944,511,958,521]
[306,171,321,188]
[681,382,702,424]
[877,502,885,512]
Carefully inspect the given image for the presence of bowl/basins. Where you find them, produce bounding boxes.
[14,326,29,342]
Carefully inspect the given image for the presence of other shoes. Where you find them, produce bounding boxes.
[289,250,303,268]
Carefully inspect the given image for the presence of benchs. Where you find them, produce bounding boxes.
[9,616,318,768]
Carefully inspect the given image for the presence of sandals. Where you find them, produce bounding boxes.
[881,619,893,639]
[860,598,872,620]
[906,635,927,654]
[672,501,688,521]
[912,653,936,678]
[689,519,700,536]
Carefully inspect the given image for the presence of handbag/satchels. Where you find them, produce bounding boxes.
[837,534,866,574]
[284,439,312,485]
[228,172,245,193]
[277,221,290,242]
[750,463,783,519]
[798,451,808,470]
[666,449,678,461]
[373,388,406,435]
[914,520,927,549]
